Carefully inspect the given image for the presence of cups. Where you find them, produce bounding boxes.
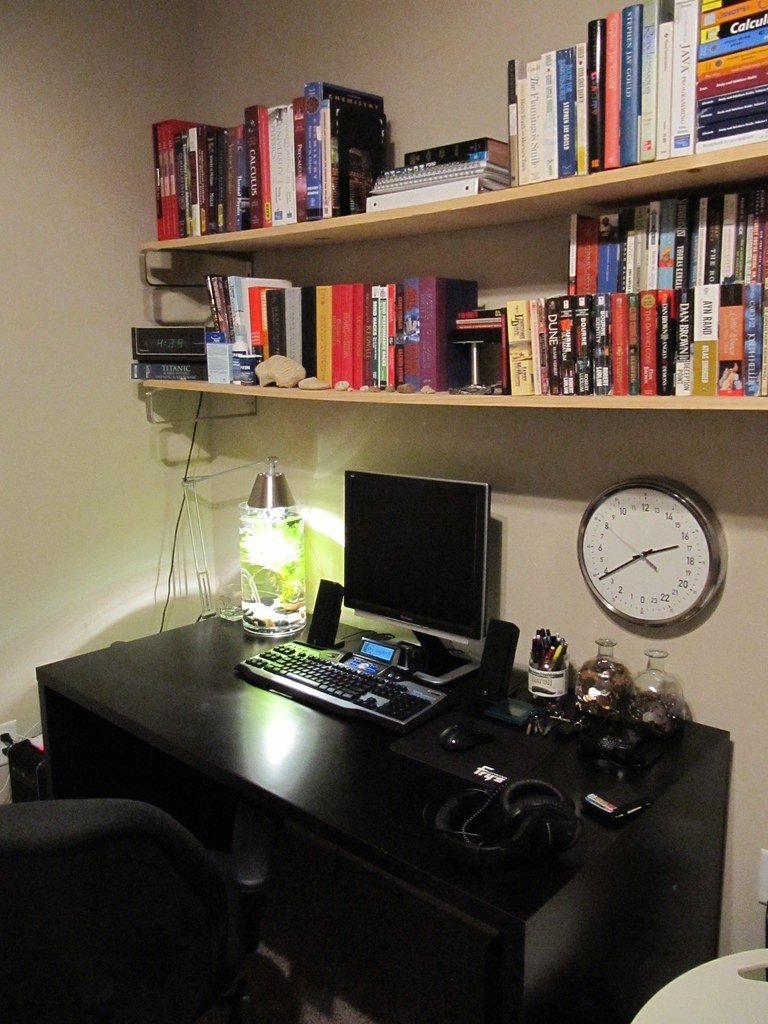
[528,652,569,700]
[238,355,261,386]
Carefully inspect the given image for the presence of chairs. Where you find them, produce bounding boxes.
[0,795,266,1024]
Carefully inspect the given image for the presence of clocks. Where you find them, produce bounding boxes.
[575,482,727,633]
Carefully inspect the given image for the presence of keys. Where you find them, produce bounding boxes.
[526,713,560,736]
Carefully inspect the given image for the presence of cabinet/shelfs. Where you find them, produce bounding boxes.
[137,144,768,422]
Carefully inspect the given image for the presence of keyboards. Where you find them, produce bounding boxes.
[233,639,449,734]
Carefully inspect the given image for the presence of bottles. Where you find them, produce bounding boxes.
[574,638,632,720]
[231,336,248,384]
[624,651,685,732]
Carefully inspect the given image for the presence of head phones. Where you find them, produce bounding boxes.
[433,777,580,858]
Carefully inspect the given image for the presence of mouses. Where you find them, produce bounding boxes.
[440,721,495,750]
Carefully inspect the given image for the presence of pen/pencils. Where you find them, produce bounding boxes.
[528,627,569,672]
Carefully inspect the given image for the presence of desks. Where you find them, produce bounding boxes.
[34,620,734,1024]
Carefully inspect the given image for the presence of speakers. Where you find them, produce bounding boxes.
[475,618,519,703]
[307,580,344,649]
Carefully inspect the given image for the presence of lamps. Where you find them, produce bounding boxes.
[181,455,296,619]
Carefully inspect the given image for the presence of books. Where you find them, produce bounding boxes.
[130,0,768,398]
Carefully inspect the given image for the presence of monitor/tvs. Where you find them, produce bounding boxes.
[344,471,491,684]
